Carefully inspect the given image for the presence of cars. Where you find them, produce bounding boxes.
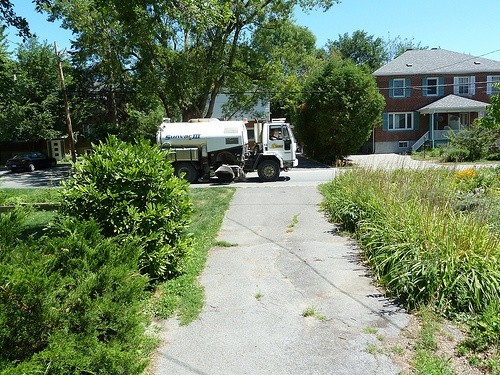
[6,152,57,173]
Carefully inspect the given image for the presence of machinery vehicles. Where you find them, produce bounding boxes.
[156,112,299,184]
